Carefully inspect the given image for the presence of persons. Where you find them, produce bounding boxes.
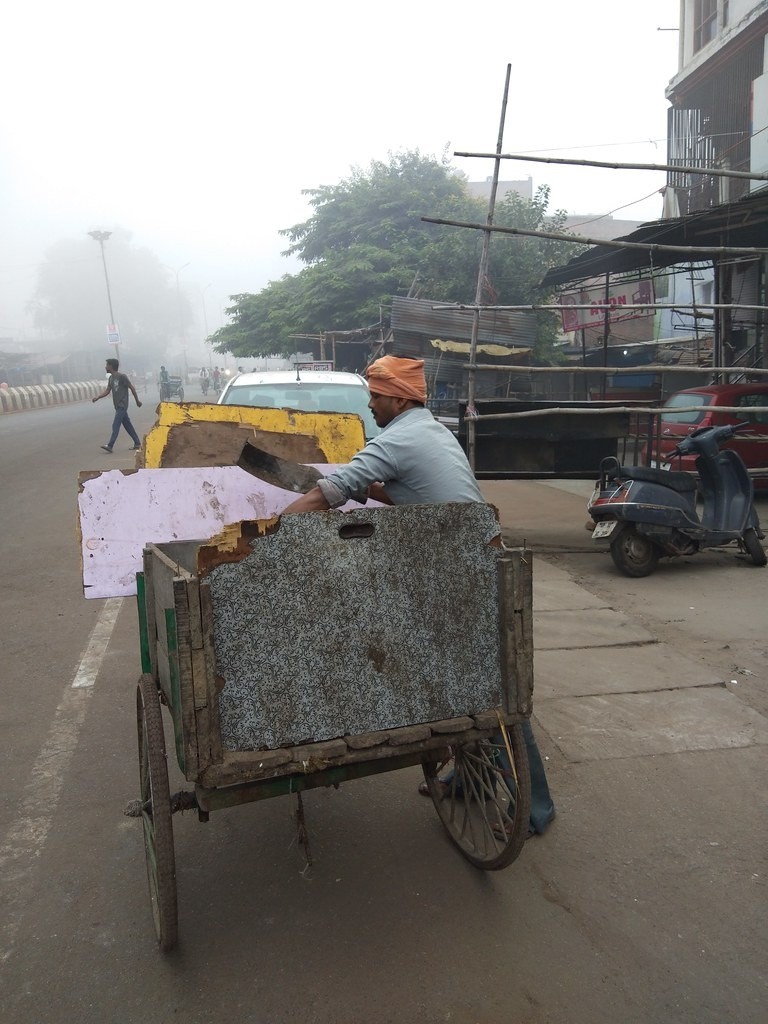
[92,358,257,453]
[280,353,556,842]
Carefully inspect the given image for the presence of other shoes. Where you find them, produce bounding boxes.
[493,808,556,841]
[101,445,112,453]
[128,445,142,450]
[418,778,498,801]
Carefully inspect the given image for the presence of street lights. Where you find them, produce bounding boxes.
[87,223,123,364]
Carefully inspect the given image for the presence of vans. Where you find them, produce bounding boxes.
[639,383,768,494]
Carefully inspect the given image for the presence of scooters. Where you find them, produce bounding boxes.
[582,421,768,578]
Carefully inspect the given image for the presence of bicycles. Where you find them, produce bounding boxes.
[201,377,209,397]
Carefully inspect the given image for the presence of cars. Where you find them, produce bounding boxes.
[213,367,383,443]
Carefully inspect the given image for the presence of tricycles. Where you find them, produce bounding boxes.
[157,376,184,401]
[120,500,538,954]
